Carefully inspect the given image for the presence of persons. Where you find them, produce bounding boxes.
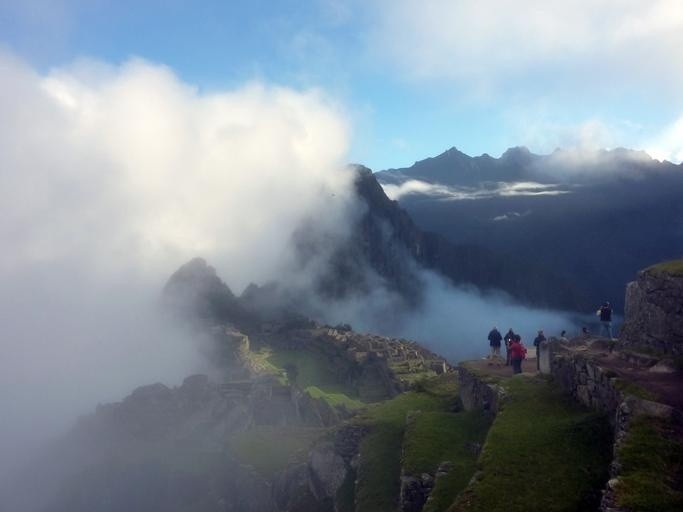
[503,328,514,366]
[596,301,613,338]
[506,334,522,374]
[487,326,502,366]
[559,330,568,343]
[533,329,546,370]
